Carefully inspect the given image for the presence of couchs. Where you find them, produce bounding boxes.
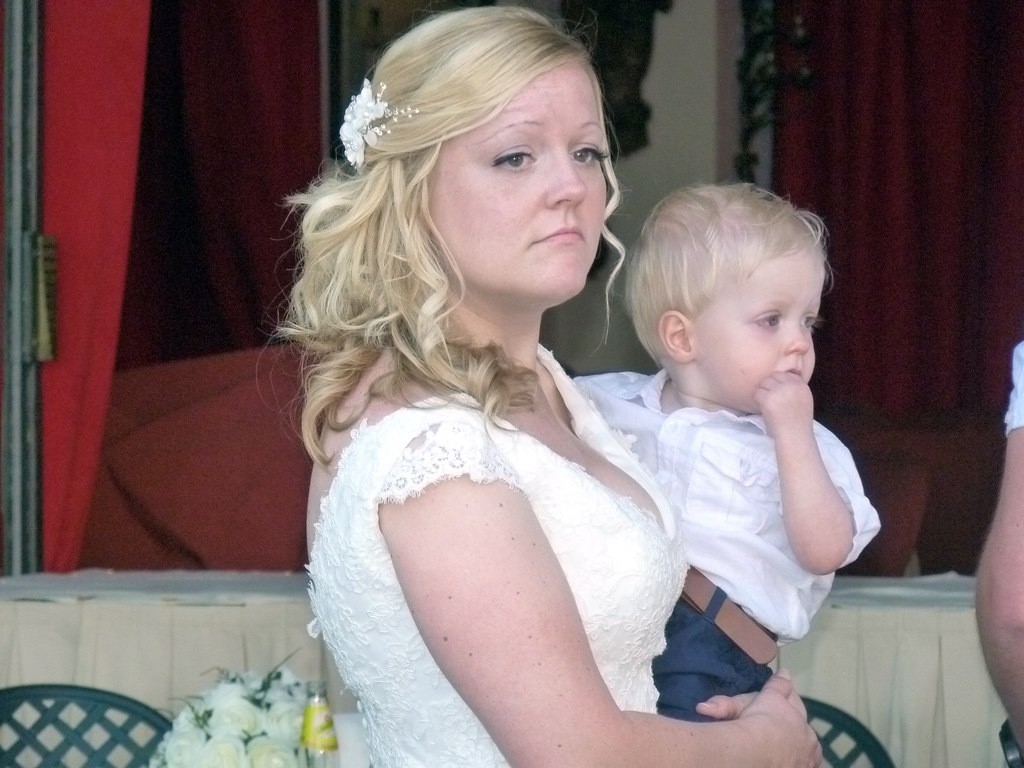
[79,345,313,575]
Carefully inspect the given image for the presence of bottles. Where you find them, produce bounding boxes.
[297,681,341,768]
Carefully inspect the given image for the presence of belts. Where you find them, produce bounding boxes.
[682,564,779,665]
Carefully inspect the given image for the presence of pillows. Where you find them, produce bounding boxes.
[104,356,312,572]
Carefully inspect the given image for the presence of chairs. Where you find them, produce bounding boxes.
[799,696,895,768]
[0,684,171,768]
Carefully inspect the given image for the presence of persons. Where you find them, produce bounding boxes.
[570,182,881,721]
[267,6,823,768]
[974,342,1024,753]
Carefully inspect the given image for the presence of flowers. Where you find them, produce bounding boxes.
[143,648,310,768]
